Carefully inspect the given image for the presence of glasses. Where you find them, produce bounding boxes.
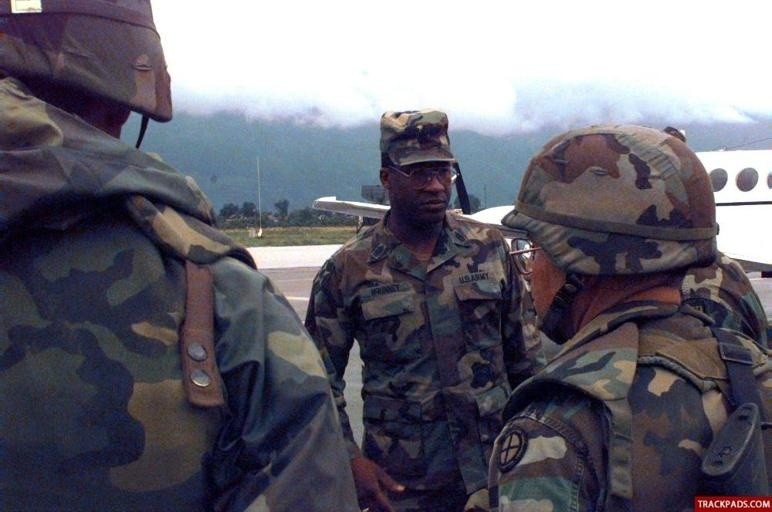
[510,238,543,275]
[386,168,460,187]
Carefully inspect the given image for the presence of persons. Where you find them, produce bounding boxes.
[0,0,365,511]
[489,121,771,509]
[303,108,546,509]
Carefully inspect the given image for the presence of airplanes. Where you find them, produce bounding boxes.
[314,148,772,278]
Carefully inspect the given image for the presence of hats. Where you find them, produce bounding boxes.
[378,109,456,169]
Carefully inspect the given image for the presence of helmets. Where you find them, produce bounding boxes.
[0,1,172,123]
[501,124,717,278]
